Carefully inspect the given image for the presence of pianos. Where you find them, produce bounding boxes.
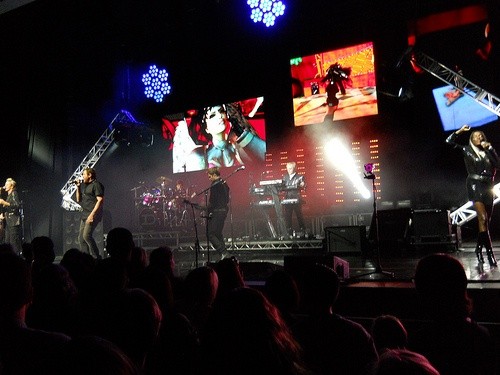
[248,178,306,241]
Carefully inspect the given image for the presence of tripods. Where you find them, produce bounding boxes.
[350,179,396,280]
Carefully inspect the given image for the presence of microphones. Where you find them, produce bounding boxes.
[72,180,83,184]
[1,186,6,189]
[237,166,245,171]
[352,241,356,245]
[480,142,493,150]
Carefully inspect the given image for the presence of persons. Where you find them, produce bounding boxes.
[0,227,500,375]
[170,179,184,208]
[74,168,104,259]
[278,161,307,240]
[200,168,230,261]
[446,125,500,267]
[172,103,266,173]
[320,62,351,122]
[0,178,23,253]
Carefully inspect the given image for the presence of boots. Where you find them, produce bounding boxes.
[475,237,485,263]
[480,230,497,266]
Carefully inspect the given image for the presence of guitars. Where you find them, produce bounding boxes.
[183,200,211,219]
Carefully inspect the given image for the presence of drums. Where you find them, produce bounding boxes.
[138,193,178,230]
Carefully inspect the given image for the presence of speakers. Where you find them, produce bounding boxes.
[324,225,369,257]
[283,255,349,281]
[368,208,450,241]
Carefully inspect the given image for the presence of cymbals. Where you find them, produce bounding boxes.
[144,186,161,191]
[153,176,174,185]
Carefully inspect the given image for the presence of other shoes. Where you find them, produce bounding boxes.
[300,232,305,239]
[283,232,293,240]
[219,251,229,260]
